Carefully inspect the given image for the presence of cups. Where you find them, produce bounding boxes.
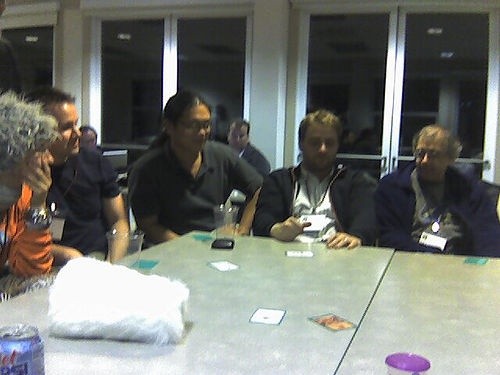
[106,229,144,273]
[212,205,239,242]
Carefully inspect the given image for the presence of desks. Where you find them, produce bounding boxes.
[333,251,500,375]
[0,229,394,375]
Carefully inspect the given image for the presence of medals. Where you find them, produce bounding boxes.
[430,220,442,233]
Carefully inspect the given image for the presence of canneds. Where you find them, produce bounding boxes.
[0,324,45,375]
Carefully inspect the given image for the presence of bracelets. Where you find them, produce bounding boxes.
[23,207,53,225]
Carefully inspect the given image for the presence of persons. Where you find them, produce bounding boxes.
[79,124,109,154]
[129,89,264,251]
[375,126,500,258]
[253,110,376,250]
[340,125,382,156]
[212,102,230,144]
[0,85,66,303]
[226,120,271,223]
[19,84,131,264]
